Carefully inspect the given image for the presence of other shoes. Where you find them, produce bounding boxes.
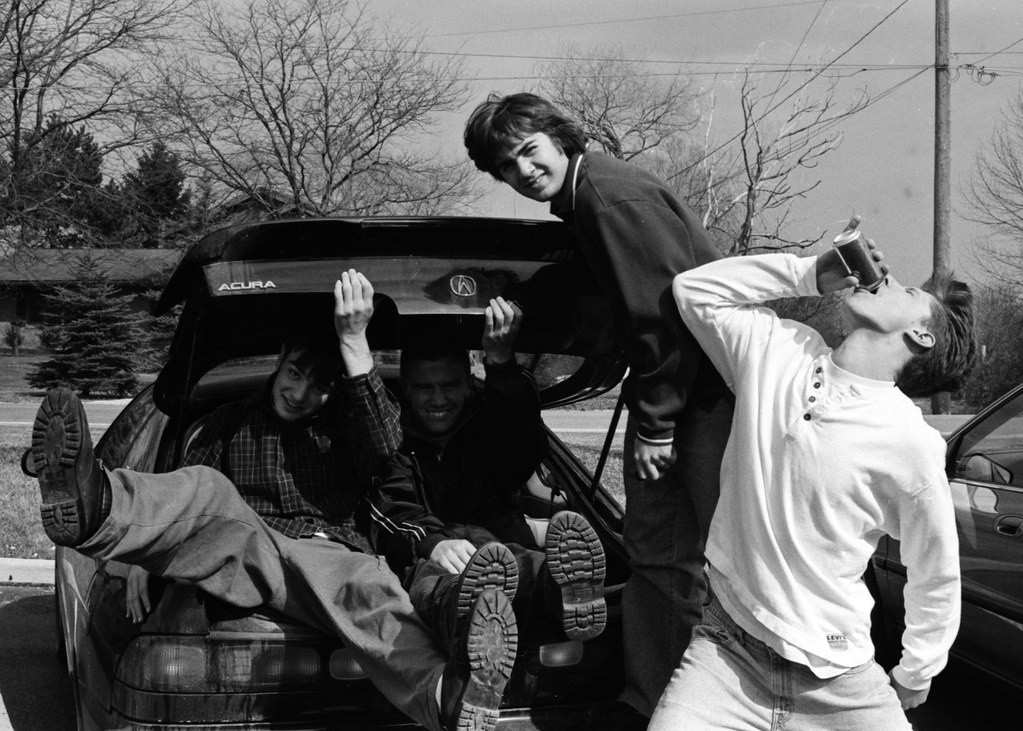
[21,388,103,545]
[546,511,607,642]
[440,588,518,731]
[458,542,519,661]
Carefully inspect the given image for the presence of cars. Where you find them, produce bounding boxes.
[864,380,1023,731]
[20,215,644,731]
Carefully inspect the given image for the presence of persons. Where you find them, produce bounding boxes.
[462,93,736,720]
[649,214,978,731]
[32,268,519,731]
[361,297,608,676]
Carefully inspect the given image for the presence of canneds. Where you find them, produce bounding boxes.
[832,228,886,292]
